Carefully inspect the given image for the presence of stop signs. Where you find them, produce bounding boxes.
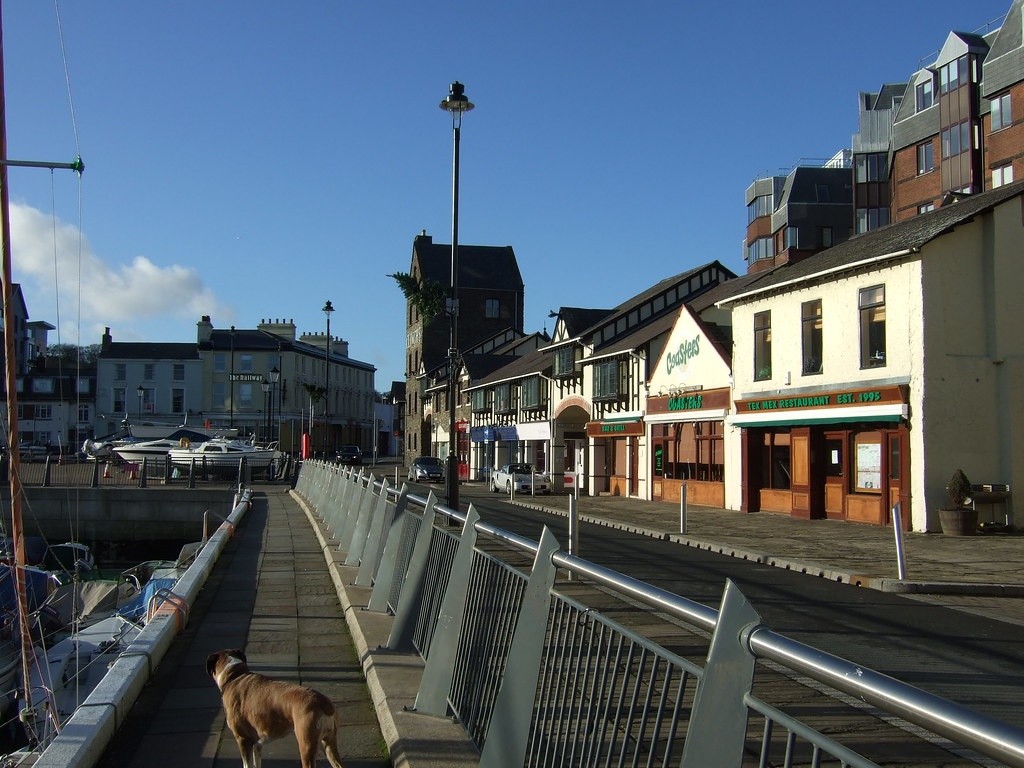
[394,431,399,437]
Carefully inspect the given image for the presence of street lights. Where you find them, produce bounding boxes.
[228,326,238,430]
[322,300,336,462]
[261,379,269,445]
[270,365,280,452]
[438,81,475,530]
[278,345,284,451]
[136,385,144,420]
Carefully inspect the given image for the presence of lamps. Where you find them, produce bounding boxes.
[657,383,688,399]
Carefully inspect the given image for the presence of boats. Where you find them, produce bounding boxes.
[4,441,50,456]
[82,413,286,476]
[0,541,177,745]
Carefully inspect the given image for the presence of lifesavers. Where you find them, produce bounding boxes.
[179,437,189,448]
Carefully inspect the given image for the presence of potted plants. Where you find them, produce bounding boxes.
[938,469,978,536]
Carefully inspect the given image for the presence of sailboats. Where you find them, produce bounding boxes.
[1,0,84,764]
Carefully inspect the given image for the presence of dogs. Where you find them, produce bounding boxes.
[206,647,342,768]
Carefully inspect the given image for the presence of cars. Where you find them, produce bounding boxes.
[408,457,446,483]
[336,446,363,463]
[492,464,552,495]
